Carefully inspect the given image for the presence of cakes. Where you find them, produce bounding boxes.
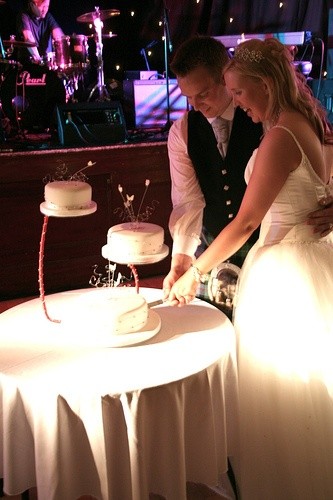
[107,222,165,256]
[79,290,148,336]
[43,181,92,210]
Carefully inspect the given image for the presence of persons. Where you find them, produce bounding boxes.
[162,36,333,323]
[168,36,333,500]
[0,0,66,139]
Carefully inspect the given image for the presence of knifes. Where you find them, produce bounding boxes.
[147,298,168,309]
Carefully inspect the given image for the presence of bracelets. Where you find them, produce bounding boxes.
[190,263,209,283]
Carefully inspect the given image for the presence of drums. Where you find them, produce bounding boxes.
[51,34,89,71]
[1,61,75,140]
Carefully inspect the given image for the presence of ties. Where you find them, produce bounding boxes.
[212,119,229,159]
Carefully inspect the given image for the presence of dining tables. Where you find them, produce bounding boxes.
[0,288,239,500]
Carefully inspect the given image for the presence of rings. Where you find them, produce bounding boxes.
[329,223,333,228]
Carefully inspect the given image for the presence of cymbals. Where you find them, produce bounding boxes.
[76,8,121,24]
[1,35,39,49]
